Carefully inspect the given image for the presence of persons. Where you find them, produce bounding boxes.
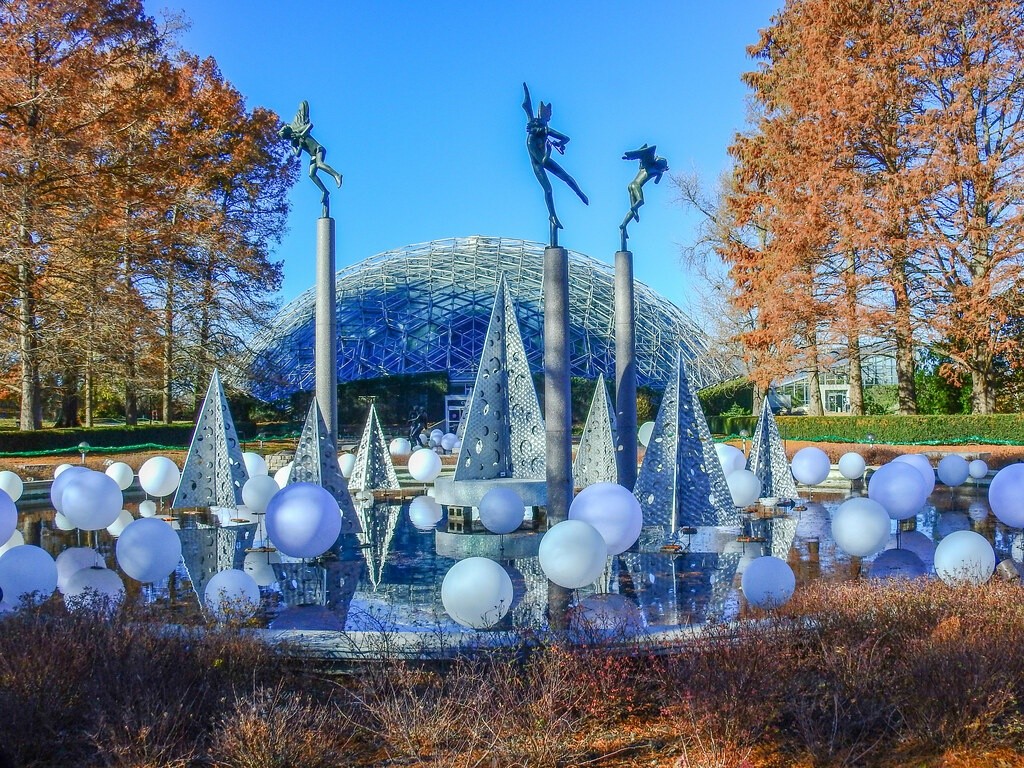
[408,405,429,449]
[618,143,668,238]
[279,100,343,204]
[521,82,588,229]
